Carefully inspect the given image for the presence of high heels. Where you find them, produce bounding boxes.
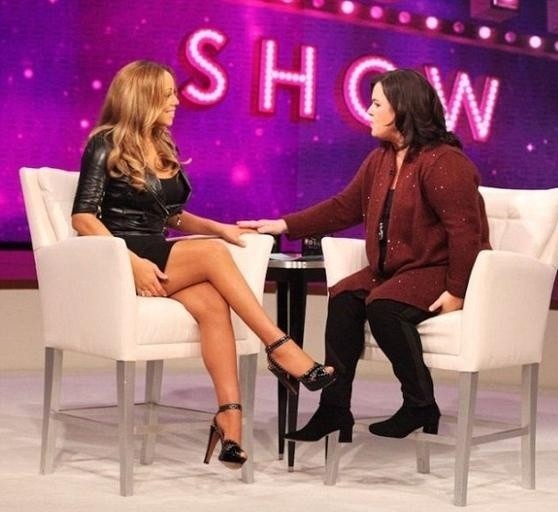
[283,405,355,444]
[204,403,247,470]
[265,335,340,399]
[370,401,441,440]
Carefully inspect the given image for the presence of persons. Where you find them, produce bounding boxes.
[234,67,494,444]
[69,58,342,465]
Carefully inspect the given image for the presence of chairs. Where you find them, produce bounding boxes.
[20,167,275,498]
[322,185,558,506]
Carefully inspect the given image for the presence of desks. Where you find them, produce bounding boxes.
[267,254,325,475]
